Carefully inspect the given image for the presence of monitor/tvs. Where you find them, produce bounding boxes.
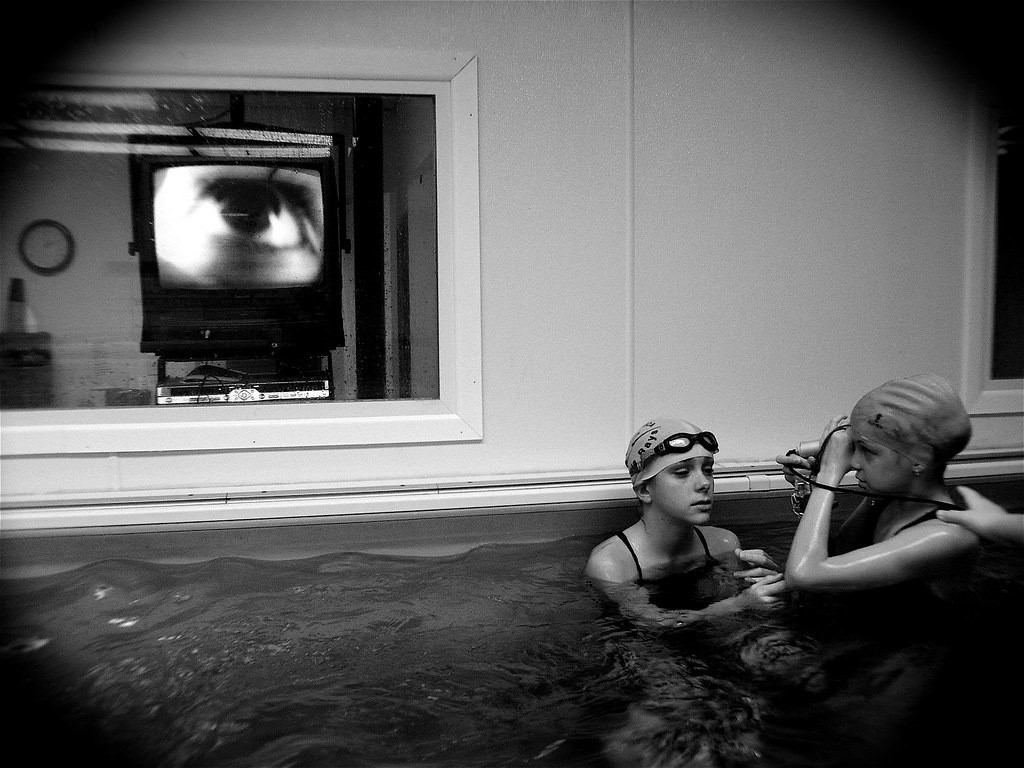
[127,135,346,304]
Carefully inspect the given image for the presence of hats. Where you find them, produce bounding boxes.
[849,372,972,476]
[625,416,713,485]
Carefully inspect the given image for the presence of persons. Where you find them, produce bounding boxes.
[585,415,786,636]
[936,485,1024,543]
[776,375,979,592]
[155,164,323,288]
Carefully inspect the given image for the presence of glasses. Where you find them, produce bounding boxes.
[629,431,720,477]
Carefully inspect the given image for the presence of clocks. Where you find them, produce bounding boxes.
[17,220,75,276]
